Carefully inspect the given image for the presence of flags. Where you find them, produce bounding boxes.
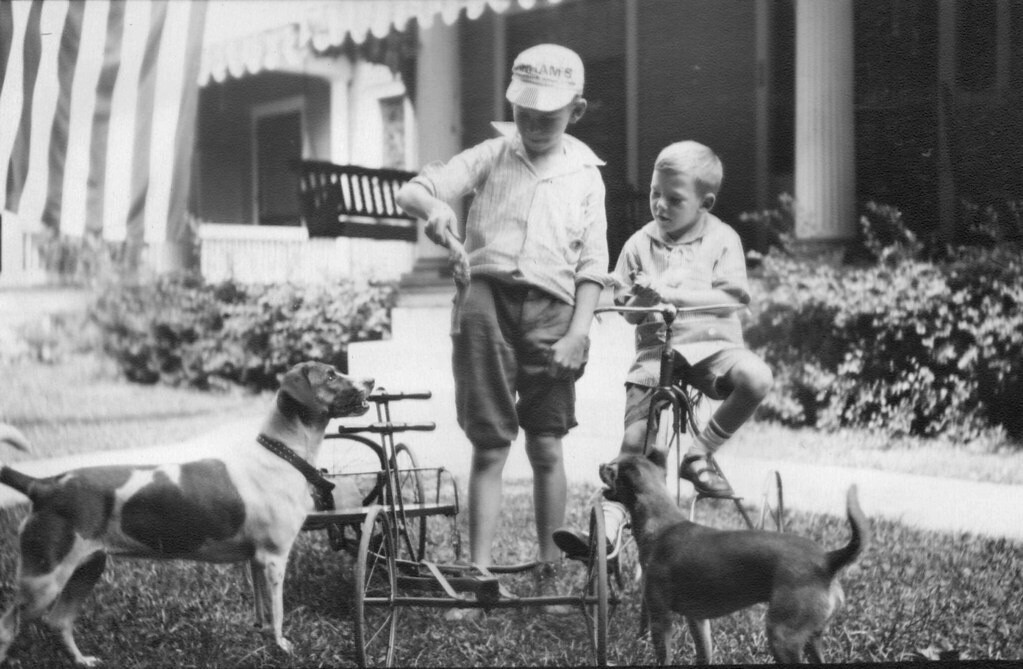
[0,0,209,242]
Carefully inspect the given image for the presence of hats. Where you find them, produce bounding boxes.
[506,45,585,112]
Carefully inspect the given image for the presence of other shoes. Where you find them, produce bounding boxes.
[443,571,477,623]
[535,552,569,615]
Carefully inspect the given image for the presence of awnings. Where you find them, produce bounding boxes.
[295,0,561,74]
[201,21,335,88]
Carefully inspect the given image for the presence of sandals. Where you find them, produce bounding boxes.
[554,525,618,572]
[679,454,732,496]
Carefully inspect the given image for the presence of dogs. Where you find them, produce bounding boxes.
[598,443,869,664]
[0,359,376,669]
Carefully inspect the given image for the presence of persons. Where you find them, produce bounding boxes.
[552,139,772,572]
[389,43,611,622]
